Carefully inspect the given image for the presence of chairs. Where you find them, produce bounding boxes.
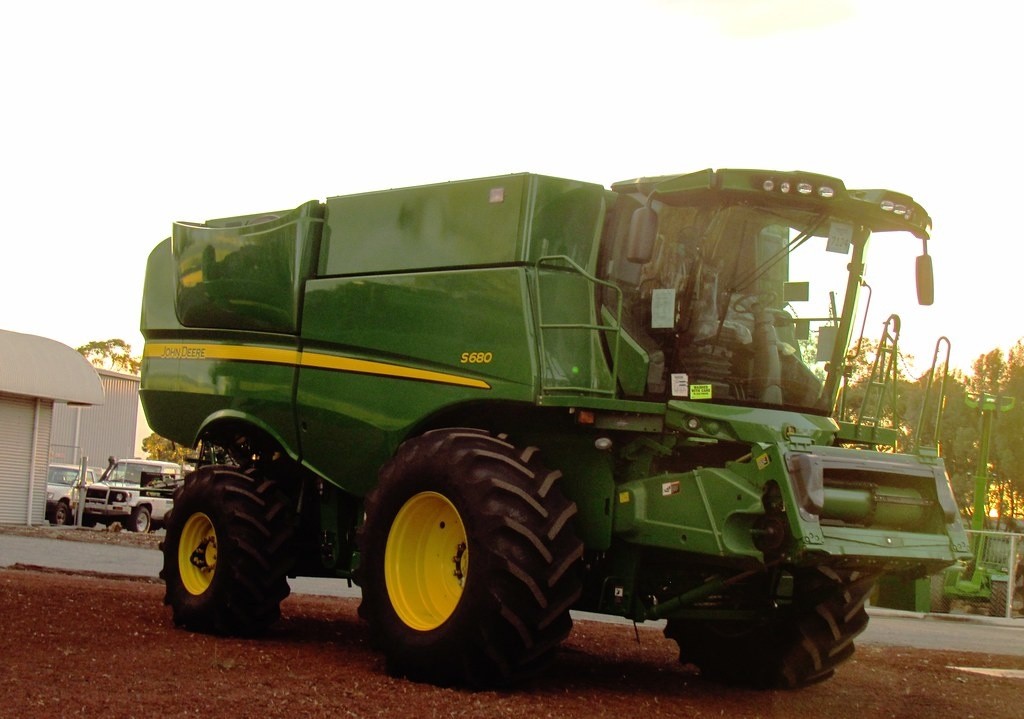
[677,252,752,400]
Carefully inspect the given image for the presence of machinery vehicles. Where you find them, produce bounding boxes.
[132,169,972,694]
[939,387,1024,618]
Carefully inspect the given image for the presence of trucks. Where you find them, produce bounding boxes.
[82,458,182,534]
[46,464,106,528]
